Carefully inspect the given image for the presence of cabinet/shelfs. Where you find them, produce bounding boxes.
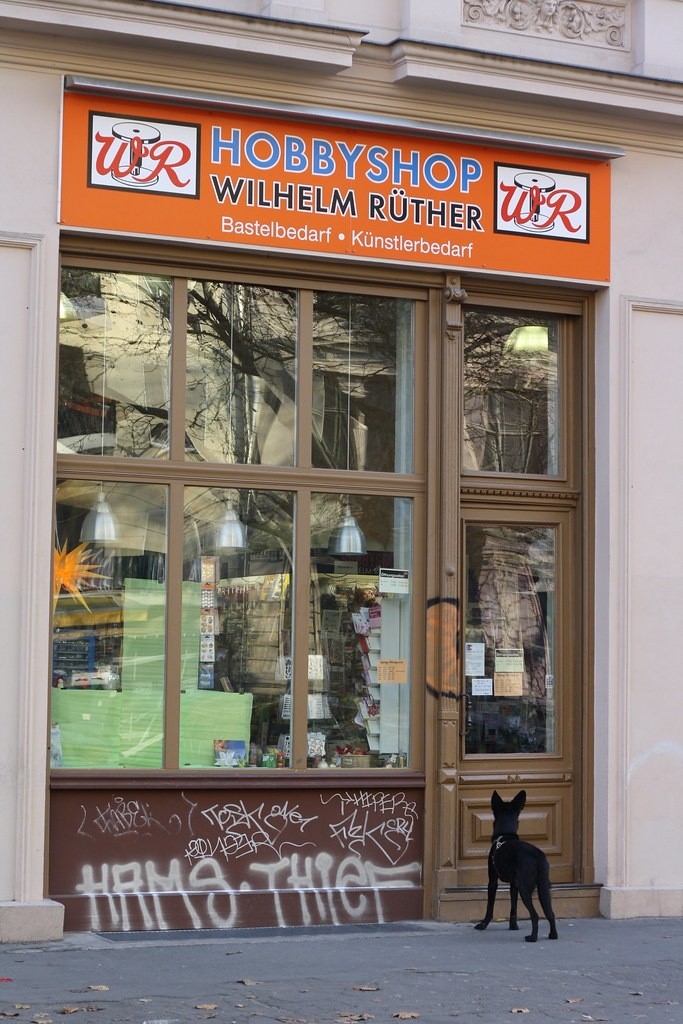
[51,628,115,692]
[215,576,286,694]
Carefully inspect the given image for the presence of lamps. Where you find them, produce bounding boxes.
[500,320,551,361]
[79,281,124,543]
[212,284,249,555]
[327,298,368,557]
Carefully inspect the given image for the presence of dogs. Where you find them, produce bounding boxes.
[474,790,559,942]
